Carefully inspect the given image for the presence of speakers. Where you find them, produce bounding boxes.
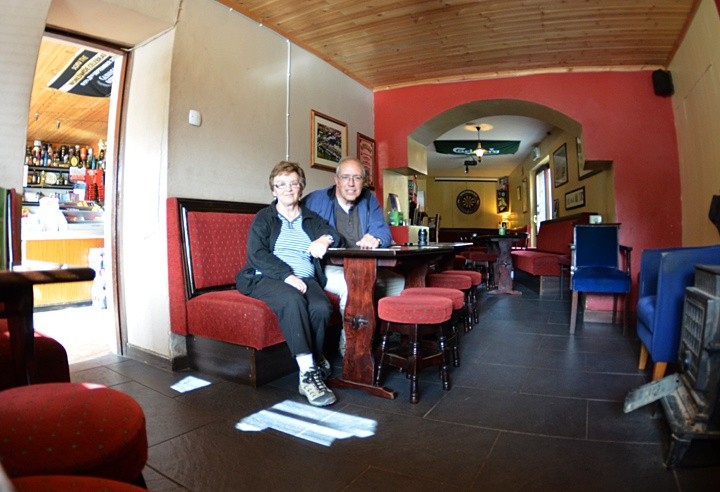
[652,68,674,97]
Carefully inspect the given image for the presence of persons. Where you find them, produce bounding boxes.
[236,160,347,407]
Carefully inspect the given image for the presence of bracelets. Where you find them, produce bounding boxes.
[300,156,405,363]
[377,238,382,244]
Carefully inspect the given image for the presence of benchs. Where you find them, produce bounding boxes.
[166,196,300,389]
[510,213,594,298]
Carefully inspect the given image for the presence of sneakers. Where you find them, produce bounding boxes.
[314,353,331,377]
[297,367,336,406]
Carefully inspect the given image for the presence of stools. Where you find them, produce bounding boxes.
[0,319,72,382]
[376,250,494,404]
[0,383,150,492]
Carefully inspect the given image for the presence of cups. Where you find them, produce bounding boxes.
[499,228,505,235]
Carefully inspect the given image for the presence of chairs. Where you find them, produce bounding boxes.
[637,245,719,381]
[568,222,634,333]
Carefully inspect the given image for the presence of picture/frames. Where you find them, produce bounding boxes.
[357,132,375,191]
[310,110,348,173]
[577,137,602,180]
[552,143,568,188]
[565,186,585,211]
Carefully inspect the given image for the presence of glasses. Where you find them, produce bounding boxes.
[272,180,301,190]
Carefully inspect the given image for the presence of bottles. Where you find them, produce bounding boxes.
[25,140,107,185]
[418,228,427,246]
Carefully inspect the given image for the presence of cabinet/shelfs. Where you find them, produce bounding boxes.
[23,168,77,204]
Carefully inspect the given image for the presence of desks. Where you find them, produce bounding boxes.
[475,236,526,298]
[0,259,95,389]
[325,242,472,401]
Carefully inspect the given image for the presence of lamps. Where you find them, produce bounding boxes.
[464,123,494,163]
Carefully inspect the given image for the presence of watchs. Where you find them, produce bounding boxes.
[323,234,334,244]
